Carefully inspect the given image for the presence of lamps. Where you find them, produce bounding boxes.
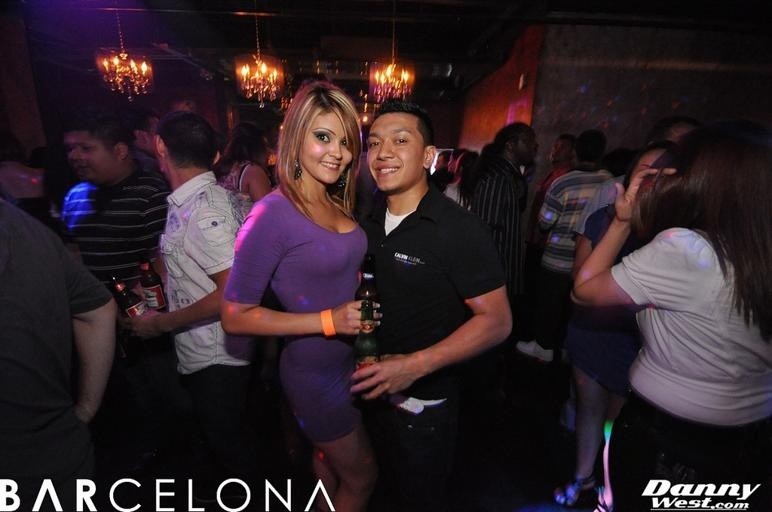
[364,18,416,104]
[231,12,286,109]
[97,3,154,105]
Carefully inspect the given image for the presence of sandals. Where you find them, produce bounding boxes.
[592,484,614,512]
[553,472,598,508]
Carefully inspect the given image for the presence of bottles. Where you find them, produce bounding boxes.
[140,256,164,308]
[353,299,383,391]
[110,276,146,318]
[353,254,379,301]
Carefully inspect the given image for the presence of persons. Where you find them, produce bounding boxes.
[1,81,771,512]
